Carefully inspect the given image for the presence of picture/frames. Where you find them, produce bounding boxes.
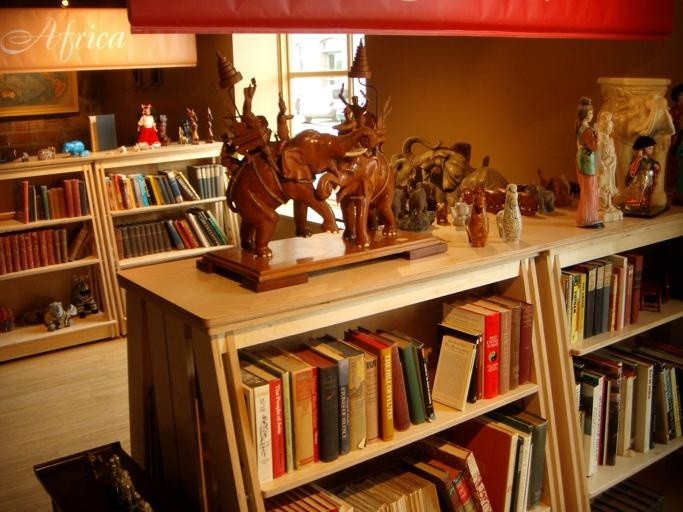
[0,67,82,119]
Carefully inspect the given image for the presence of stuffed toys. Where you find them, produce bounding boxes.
[43,298,76,333]
[69,276,98,319]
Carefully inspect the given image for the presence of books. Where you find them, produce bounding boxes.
[0,163,236,275]
[560,245,669,341]
[265,410,549,512]
[239,291,533,482]
[571,338,679,477]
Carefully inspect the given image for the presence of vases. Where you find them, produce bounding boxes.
[596,76,677,208]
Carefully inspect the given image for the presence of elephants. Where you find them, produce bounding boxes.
[225,125,379,260]
[314,146,399,250]
[402,135,509,222]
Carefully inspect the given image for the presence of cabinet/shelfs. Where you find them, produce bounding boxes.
[117,198,575,510]
[0,152,123,369]
[83,137,246,342]
[434,194,681,512]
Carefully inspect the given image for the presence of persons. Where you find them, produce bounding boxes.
[593,109,619,213]
[273,89,294,139]
[333,106,358,132]
[573,93,602,228]
[668,82,682,209]
[335,82,372,128]
[622,135,658,215]
